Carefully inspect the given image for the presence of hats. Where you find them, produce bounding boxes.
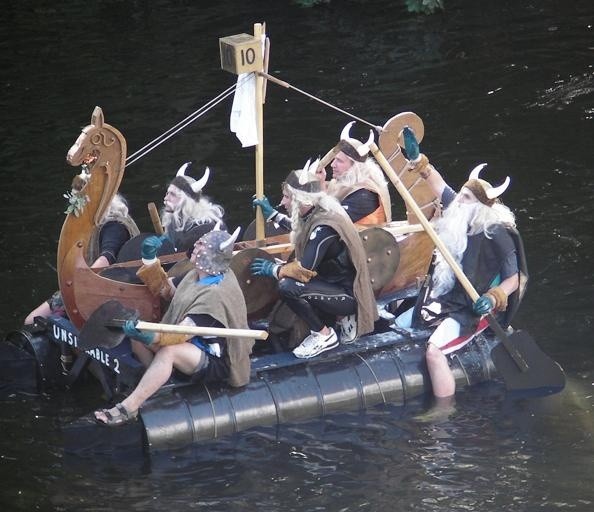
[194,230,234,277]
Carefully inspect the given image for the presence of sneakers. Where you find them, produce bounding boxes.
[338,314,359,345]
[290,325,342,361]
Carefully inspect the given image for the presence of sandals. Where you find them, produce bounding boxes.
[93,401,141,429]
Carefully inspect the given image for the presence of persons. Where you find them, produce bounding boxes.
[21,170,143,327]
[158,161,229,256]
[314,135,394,231]
[90,224,257,427]
[248,168,363,360]
[410,162,529,426]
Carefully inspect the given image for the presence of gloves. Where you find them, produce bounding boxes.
[471,295,494,317]
[249,257,280,280]
[120,319,154,346]
[139,233,164,260]
[401,125,421,162]
[252,193,279,223]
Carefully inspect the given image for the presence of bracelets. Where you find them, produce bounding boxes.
[413,151,431,175]
[487,285,508,315]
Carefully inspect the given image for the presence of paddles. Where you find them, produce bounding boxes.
[77,300,269,351]
[243,141,343,241]
[369,143,565,399]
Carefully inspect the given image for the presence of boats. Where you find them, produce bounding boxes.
[5,23,531,463]
